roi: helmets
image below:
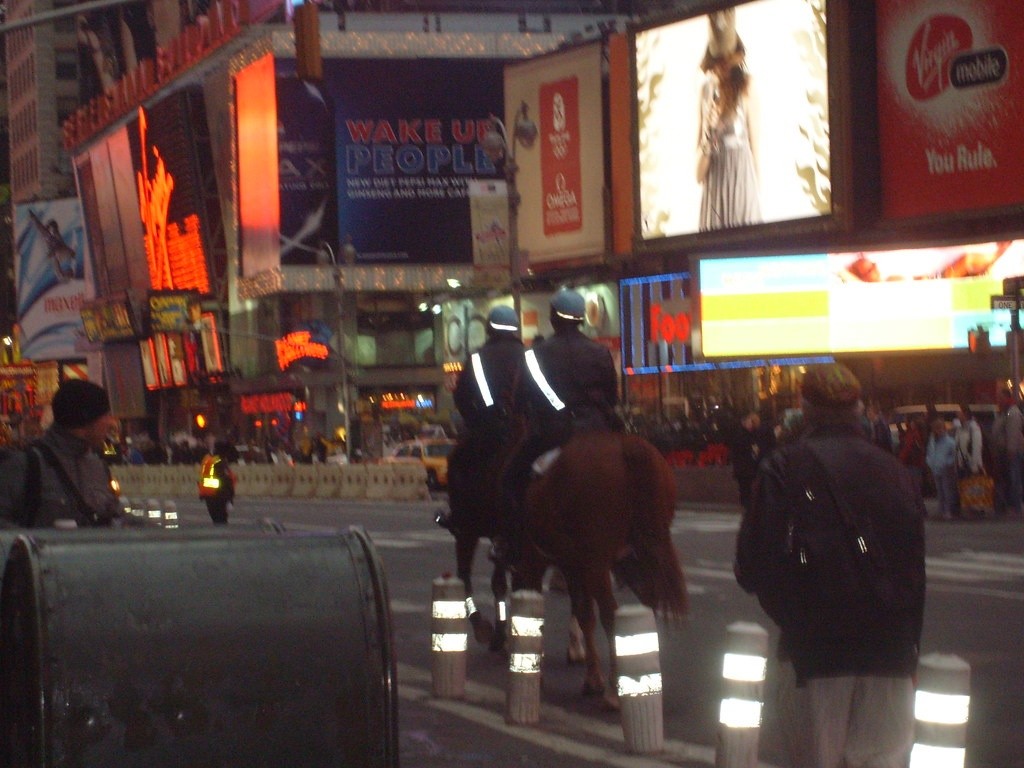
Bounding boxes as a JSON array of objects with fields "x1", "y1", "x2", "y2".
[
  {"x1": 487, "y1": 306, "x2": 519, "y2": 331},
  {"x1": 550, "y1": 291, "x2": 586, "y2": 325}
]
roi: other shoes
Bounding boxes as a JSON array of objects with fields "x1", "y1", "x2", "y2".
[{"x1": 488, "y1": 542, "x2": 519, "y2": 566}]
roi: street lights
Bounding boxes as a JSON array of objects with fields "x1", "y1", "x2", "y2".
[
  {"x1": 319, "y1": 239, "x2": 351, "y2": 464},
  {"x1": 480, "y1": 100, "x2": 539, "y2": 345}
]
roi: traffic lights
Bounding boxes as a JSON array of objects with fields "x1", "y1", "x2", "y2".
[{"x1": 969, "y1": 331, "x2": 977, "y2": 352}]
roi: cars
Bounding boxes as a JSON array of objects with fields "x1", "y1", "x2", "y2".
[{"x1": 378, "y1": 439, "x2": 457, "y2": 487}]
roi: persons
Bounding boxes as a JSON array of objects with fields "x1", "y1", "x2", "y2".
[
  {"x1": 696, "y1": 7, "x2": 760, "y2": 231},
  {"x1": 486, "y1": 297, "x2": 618, "y2": 564},
  {"x1": 198, "y1": 432, "x2": 235, "y2": 525},
  {"x1": 732, "y1": 360, "x2": 926, "y2": 768},
  {"x1": 433, "y1": 304, "x2": 525, "y2": 545},
  {"x1": 734, "y1": 390, "x2": 1024, "y2": 517},
  {"x1": 1, "y1": 378, "x2": 120, "y2": 530}
]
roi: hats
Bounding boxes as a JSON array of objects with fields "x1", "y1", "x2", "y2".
[
  {"x1": 800, "y1": 361, "x2": 861, "y2": 408},
  {"x1": 51, "y1": 379, "x2": 109, "y2": 431}
]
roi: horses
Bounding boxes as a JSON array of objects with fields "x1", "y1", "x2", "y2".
[{"x1": 446, "y1": 334, "x2": 690, "y2": 712}]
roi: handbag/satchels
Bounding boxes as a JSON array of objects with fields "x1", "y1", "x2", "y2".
[{"x1": 960, "y1": 478, "x2": 994, "y2": 513}]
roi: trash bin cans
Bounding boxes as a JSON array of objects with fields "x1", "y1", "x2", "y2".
[{"x1": 0, "y1": 525, "x2": 401, "y2": 768}]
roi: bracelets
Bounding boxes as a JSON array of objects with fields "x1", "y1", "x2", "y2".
[{"x1": 701, "y1": 130, "x2": 714, "y2": 157}]
